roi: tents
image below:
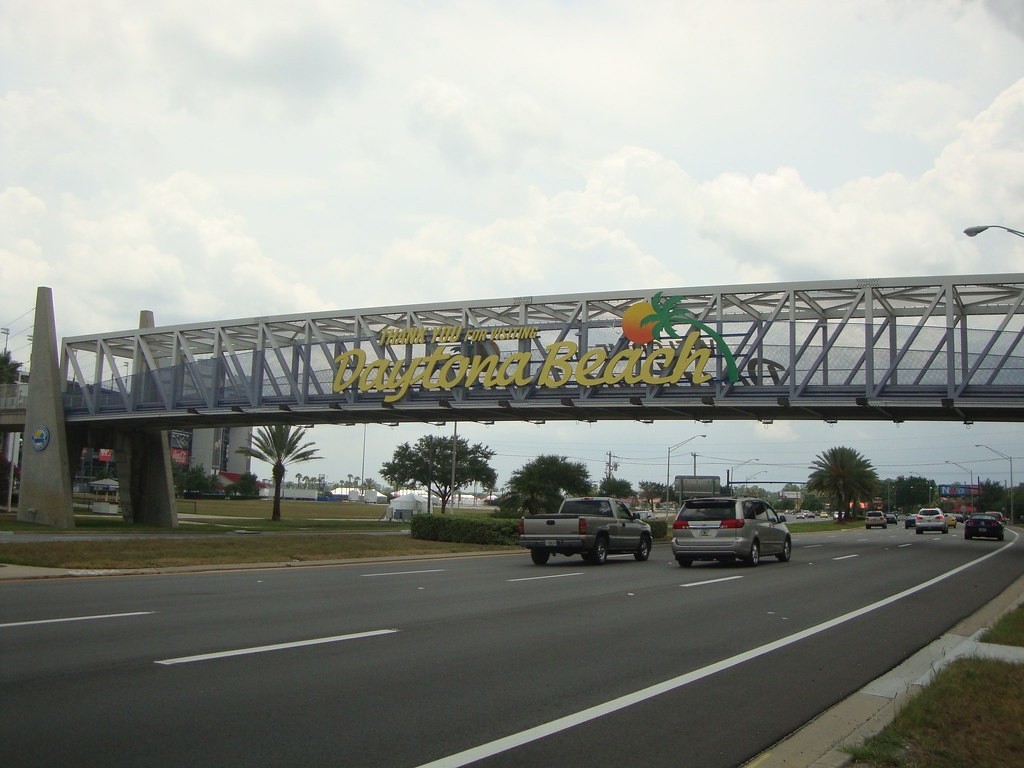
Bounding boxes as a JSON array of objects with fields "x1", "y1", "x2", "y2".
[{"x1": 388, "y1": 493, "x2": 432, "y2": 522}]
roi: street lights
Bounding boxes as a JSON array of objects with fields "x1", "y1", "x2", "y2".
[
  {"x1": 975, "y1": 444, "x2": 1013, "y2": 526},
  {"x1": 908, "y1": 471, "x2": 932, "y2": 505},
  {"x1": 745, "y1": 470, "x2": 767, "y2": 491},
  {"x1": 946, "y1": 458, "x2": 974, "y2": 516},
  {"x1": 665, "y1": 434, "x2": 707, "y2": 519},
  {"x1": 730, "y1": 458, "x2": 760, "y2": 498}
]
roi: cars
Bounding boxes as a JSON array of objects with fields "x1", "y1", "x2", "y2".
[
  {"x1": 964, "y1": 512, "x2": 1006, "y2": 540},
  {"x1": 795, "y1": 507, "x2": 964, "y2": 534}
]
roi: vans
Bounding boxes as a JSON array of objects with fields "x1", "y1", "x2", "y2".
[
  {"x1": 986, "y1": 511, "x2": 1005, "y2": 528},
  {"x1": 671, "y1": 494, "x2": 792, "y2": 567}
]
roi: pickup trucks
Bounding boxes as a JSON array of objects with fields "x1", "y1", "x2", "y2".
[{"x1": 517, "y1": 496, "x2": 653, "y2": 564}]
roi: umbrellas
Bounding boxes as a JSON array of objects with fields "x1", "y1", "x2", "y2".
[{"x1": 87, "y1": 477, "x2": 119, "y2": 502}]
